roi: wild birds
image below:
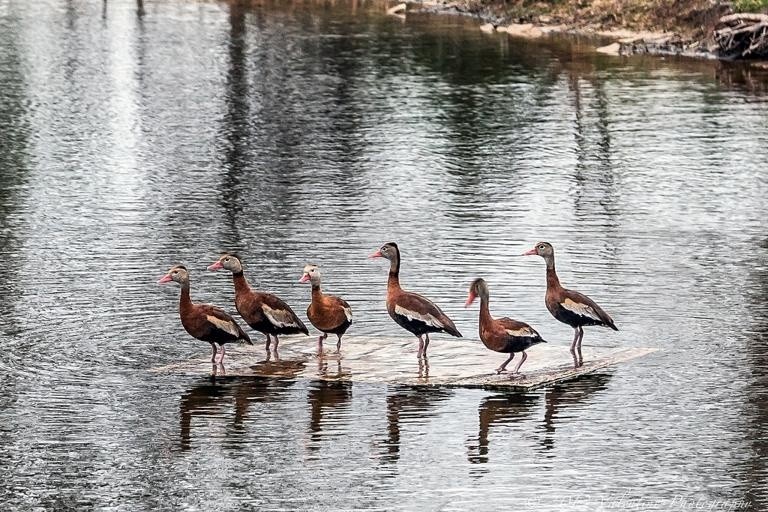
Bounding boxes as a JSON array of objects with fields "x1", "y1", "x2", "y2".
[
  {"x1": 367, "y1": 242, "x2": 463, "y2": 358},
  {"x1": 521, "y1": 242, "x2": 619, "y2": 350},
  {"x1": 157, "y1": 265, "x2": 254, "y2": 362},
  {"x1": 298, "y1": 266, "x2": 353, "y2": 352},
  {"x1": 464, "y1": 277, "x2": 547, "y2": 374},
  {"x1": 206, "y1": 254, "x2": 310, "y2": 352}
]
